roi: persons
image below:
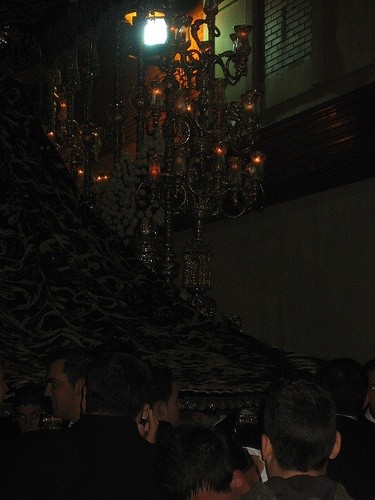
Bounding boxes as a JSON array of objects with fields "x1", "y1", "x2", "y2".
[
  {"x1": 0, "y1": 348, "x2": 178, "y2": 500},
  {"x1": 239, "y1": 374, "x2": 354, "y2": 500},
  {"x1": 317, "y1": 357, "x2": 375, "y2": 500},
  {"x1": 363, "y1": 358, "x2": 375, "y2": 423},
  {"x1": 0, "y1": 362, "x2": 9, "y2": 402},
  {"x1": 158, "y1": 426, "x2": 259, "y2": 500},
  {"x1": 42, "y1": 348, "x2": 86, "y2": 421},
  {"x1": 141, "y1": 366, "x2": 180, "y2": 445},
  {"x1": 13, "y1": 382, "x2": 52, "y2": 432}
]
